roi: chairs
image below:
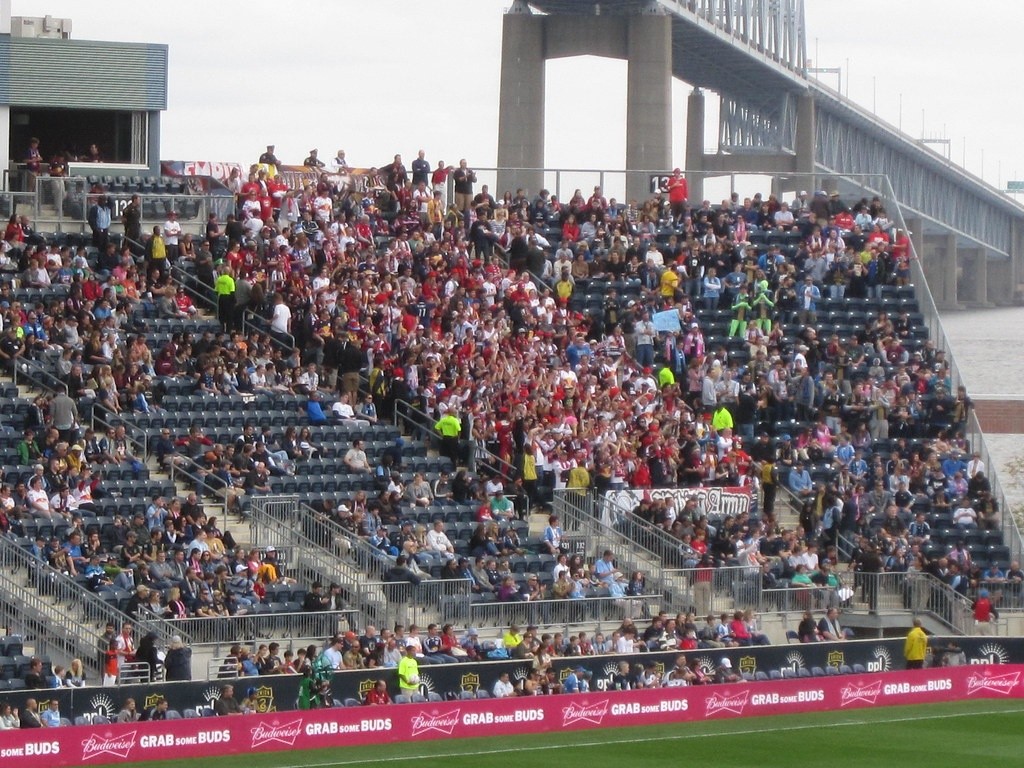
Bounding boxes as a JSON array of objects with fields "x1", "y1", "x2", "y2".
[{"x1": 0, "y1": 170, "x2": 1024, "y2": 730}]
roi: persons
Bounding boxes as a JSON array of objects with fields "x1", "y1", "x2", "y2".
[{"x1": 0, "y1": 143, "x2": 1022, "y2": 733}]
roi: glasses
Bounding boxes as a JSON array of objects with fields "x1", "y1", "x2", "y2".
[
  {"x1": 163, "y1": 433, "x2": 170, "y2": 435},
  {"x1": 139, "y1": 518, "x2": 145, "y2": 520}
]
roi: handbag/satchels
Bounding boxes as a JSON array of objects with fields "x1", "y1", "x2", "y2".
[{"x1": 155, "y1": 358, "x2": 172, "y2": 375}]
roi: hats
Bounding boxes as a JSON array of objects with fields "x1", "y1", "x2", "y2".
[
  {"x1": 236, "y1": 565, "x2": 248, "y2": 573},
  {"x1": 81, "y1": 464, "x2": 93, "y2": 470},
  {"x1": 71, "y1": 445, "x2": 83, "y2": 451},
  {"x1": 265, "y1": 546, "x2": 278, "y2": 552},
  {"x1": 783, "y1": 435, "x2": 791, "y2": 440},
  {"x1": 417, "y1": 325, "x2": 427, "y2": 330},
  {"x1": 830, "y1": 191, "x2": 839, "y2": 196},
  {"x1": 576, "y1": 667, "x2": 587, "y2": 671},
  {"x1": 467, "y1": 628, "x2": 478, "y2": 636},
  {"x1": 799, "y1": 345, "x2": 809, "y2": 351},
  {"x1": 798, "y1": 191, "x2": 808, "y2": 196},
  {"x1": 137, "y1": 585, "x2": 149, "y2": 592},
  {"x1": 2, "y1": 301, "x2": 11, "y2": 308},
  {"x1": 722, "y1": 658, "x2": 732, "y2": 668},
  {"x1": 818, "y1": 191, "x2": 827, "y2": 196},
  {"x1": 126, "y1": 531, "x2": 139, "y2": 536},
  {"x1": 310, "y1": 148, "x2": 318, "y2": 153}
]
